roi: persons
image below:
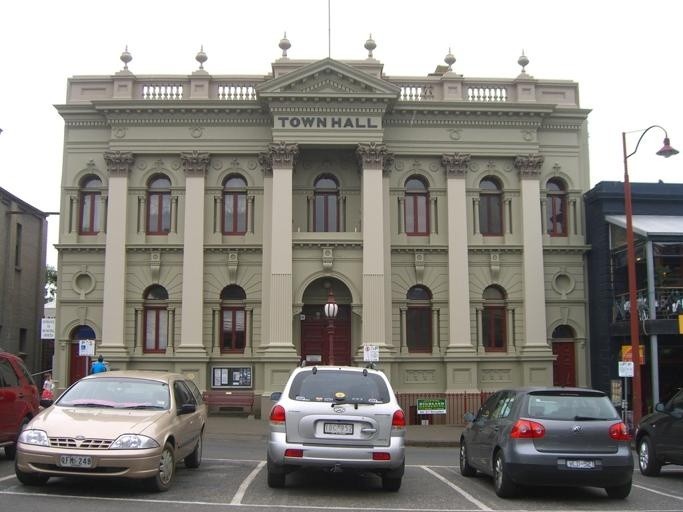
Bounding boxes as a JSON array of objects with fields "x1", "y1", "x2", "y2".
[
  {"x1": 90, "y1": 354, "x2": 107, "y2": 374},
  {"x1": 41, "y1": 373, "x2": 55, "y2": 400}
]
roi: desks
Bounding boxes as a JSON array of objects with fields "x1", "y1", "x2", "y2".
[{"x1": 624, "y1": 298, "x2": 659, "y2": 319}]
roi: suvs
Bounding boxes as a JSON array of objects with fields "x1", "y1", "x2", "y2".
[
  {"x1": 267, "y1": 359, "x2": 408, "y2": 490},
  {"x1": 0, "y1": 350, "x2": 37, "y2": 460}
]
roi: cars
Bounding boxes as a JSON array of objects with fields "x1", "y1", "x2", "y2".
[
  {"x1": 457, "y1": 388, "x2": 633, "y2": 501},
  {"x1": 637, "y1": 389, "x2": 683, "y2": 478},
  {"x1": 14, "y1": 369, "x2": 209, "y2": 494}
]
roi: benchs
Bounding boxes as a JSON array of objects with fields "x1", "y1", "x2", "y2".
[{"x1": 202, "y1": 391, "x2": 255, "y2": 414}]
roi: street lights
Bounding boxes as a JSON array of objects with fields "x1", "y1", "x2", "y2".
[
  {"x1": 321, "y1": 292, "x2": 341, "y2": 365},
  {"x1": 621, "y1": 123, "x2": 681, "y2": 436}
]
